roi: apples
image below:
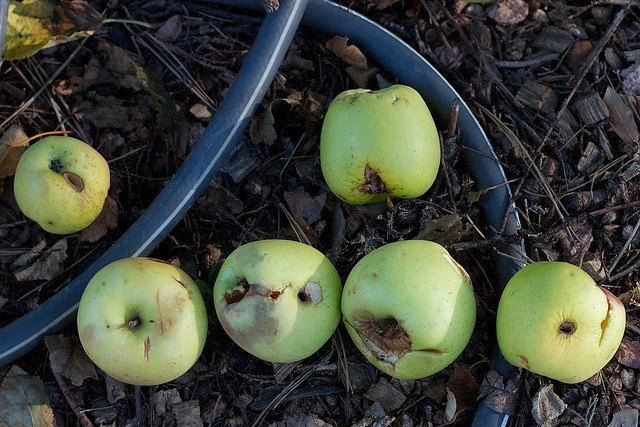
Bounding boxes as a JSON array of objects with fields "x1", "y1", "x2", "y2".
[
  {"x1": 212, "y1": 239, "x2": 344, "y2": 363},
  {"x1": 496, "y1": 260, "x2": 627, "y2": 385},
  {"x1": 340, "y1": 239, "x2": 477, "y2": 380},
  {"x1": 77, "y1": 258, "x2": 208, "y2": 386},
  {"x1": 319, "y1": 84, "x2": 441, "y2": 205},
  {"x1": 14, "y1": 136, "x2": 111, "y2": 235}
]
